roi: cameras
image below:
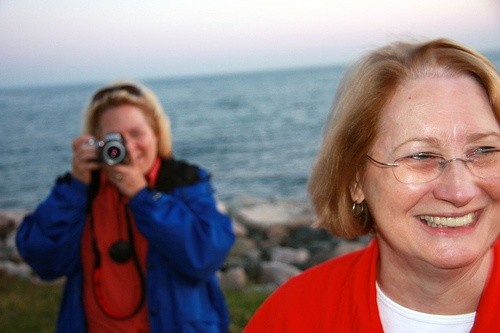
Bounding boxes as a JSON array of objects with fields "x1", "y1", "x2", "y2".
[{"x1": 84, "y1": 132, "x2": 130, "y2": 167}]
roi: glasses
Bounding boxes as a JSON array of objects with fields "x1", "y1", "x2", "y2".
[
  {"x1": 363, "y1": 147, "x2": 500, "y2": 184},
  {"x1": 91, "y1": 86, "x2": 141, "y2": 105}
]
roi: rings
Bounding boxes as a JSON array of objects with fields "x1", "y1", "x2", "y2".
[{"x1": 114, "y1": 172, "x2": 121, "y2": 180}]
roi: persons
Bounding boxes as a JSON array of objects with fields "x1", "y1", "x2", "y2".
[
  {"x1": 240, "y1": 36, "x2": 500, "y2": 333},
  {"x1": 15, "y1": 82, "x2": 235, "y2": 333}
]
